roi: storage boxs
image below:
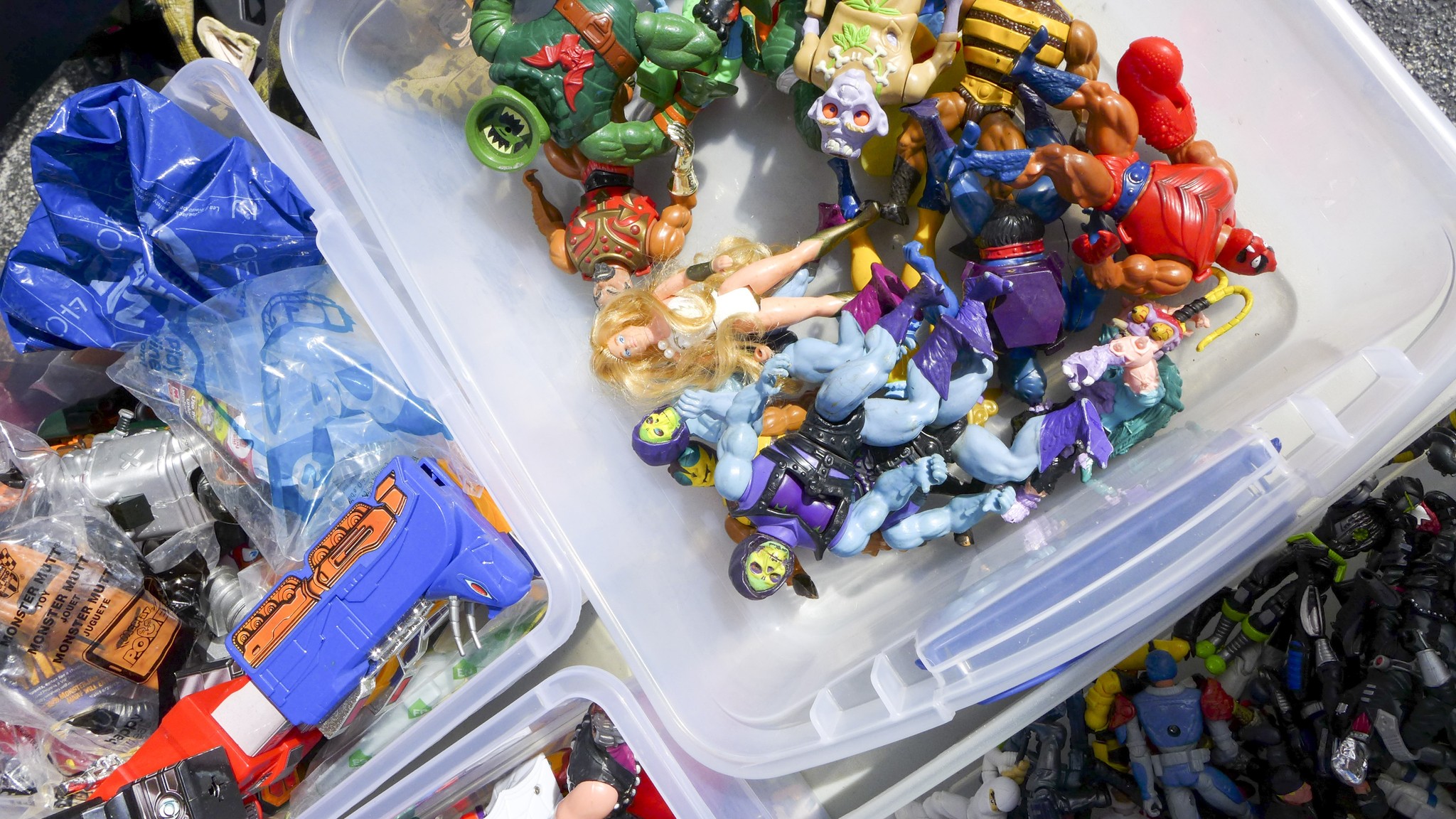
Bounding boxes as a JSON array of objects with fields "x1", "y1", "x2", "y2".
[{"x1": 0, "y1": 0, "x2": 1456, "y2": 819}]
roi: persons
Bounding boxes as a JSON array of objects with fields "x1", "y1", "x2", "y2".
[
  {"x1": 895, "y1": 411, "x2": 1456, "y2": 819},
  {"x1": 471, "y1": 1, "x2": 1278, "y2": 601}
]
